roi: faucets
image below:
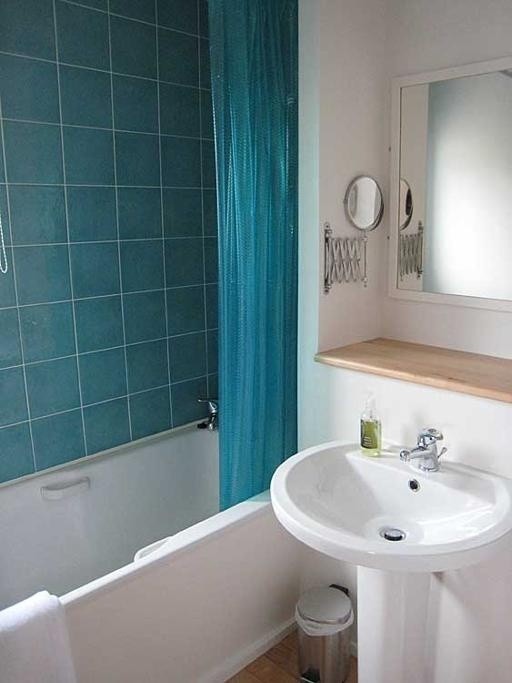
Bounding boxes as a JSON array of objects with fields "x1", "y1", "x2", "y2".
[
  {"x1": 197, "y1": 398, "x2": 219, "y2": 432},
  {"x1": 401, "y1": 428, "x2": 443, "y2": 471}
]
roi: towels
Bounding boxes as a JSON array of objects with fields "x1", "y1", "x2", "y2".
[{"x1": 0, "y1": 586, "x2": 81, "y2": 683}]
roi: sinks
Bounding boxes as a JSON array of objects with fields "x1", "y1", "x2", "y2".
[{"x1": 270, "y1": 437, "x2": 512, "y2": 574}]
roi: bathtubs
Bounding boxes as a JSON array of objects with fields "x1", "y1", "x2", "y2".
[{"x1": 0, "y1": 413, "x2": 299, "y2": 683}]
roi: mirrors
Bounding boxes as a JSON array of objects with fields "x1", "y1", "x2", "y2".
[
  {"x1": 386, "y1": 51, "x2": 511, "y2": 308},
  {"x1": 399, "y1": 177, "x2": 427, "y2": 283},
  {"x1": 321, "y1": 174, "x2": 387, "y2": 296}
]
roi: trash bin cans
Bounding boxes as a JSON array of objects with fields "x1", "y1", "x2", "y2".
[{"x1": 296, "y1": 585, "x2": 355, "y2": 683}]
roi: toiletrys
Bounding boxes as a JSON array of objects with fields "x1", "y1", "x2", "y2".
[{"x1": 360, "y1": 395, "x2": 382, "y2": 457}]
